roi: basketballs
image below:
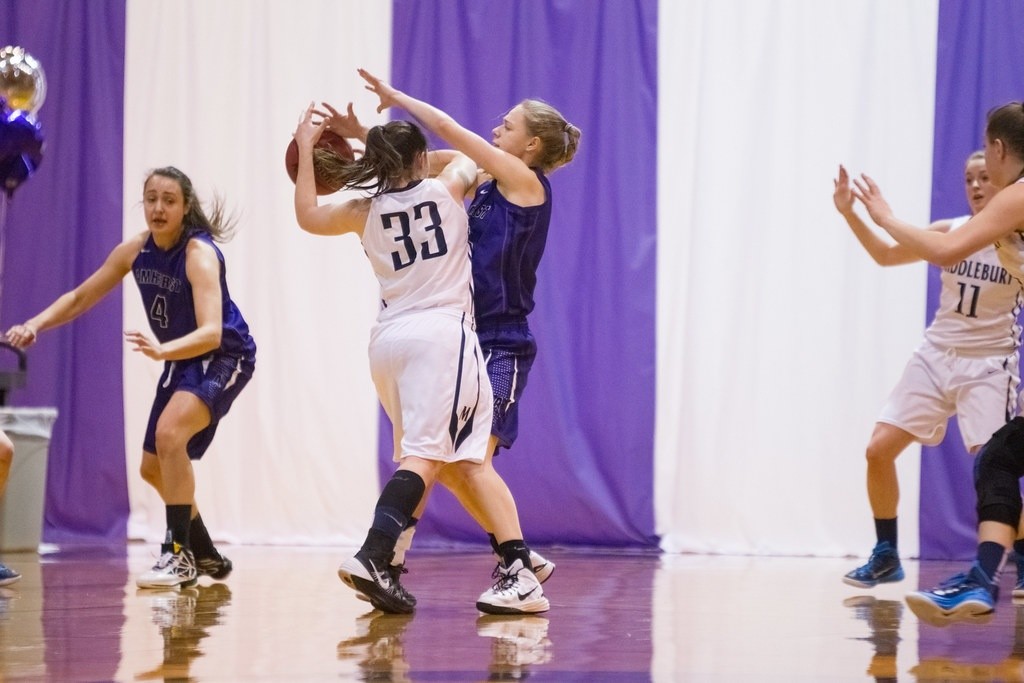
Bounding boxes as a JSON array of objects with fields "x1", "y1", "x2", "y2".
[{"x1": 284, "y1": 128, "x2": 355, "y2": 199}]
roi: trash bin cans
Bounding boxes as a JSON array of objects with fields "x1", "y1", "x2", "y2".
[{"x1": 0, "y1": 406, "x2": 58, "y2": 552}]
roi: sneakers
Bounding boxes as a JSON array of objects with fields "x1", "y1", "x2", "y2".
[
  {"x1": 1012, "y1": 551, "x2": 1024, "y2": 597},
  {"x1": 905, "y1": 560, "x2": 1001, "y2": 628},
  {"x1": 1, "y1": 565, "x2": 21, "y2": 587},
  {"x1": 181, "y1": 553, "x2": 233, "y2": 589},
  {"x1": 843, "y1": 541, "x2": 905, "y2": 589},
  {"x1": 136, "y1": 543, "x2": 199, "y2": 590},
  {"x1": 338, "y1": 551, "x2": 417, "y2": 614},
  {"x1": 354, "y1": 592, "x2": 370, "y2": 601},
  {"x1": 475, "y1": 558, "x2": 550, "y2": 615},
  {"x1": 529, "y1": 550, "x2": 556, "y2": 583}
]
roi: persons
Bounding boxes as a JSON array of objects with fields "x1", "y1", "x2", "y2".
[
  {"x1": 290, "y1": 102, "x2": 493, "y2": 613},
  {"x1": 0, "y1": 428, "x2": 22, "y2": 587},
  {"x1": 310, "y1": 67, "x2": 581, "y2": 614},
  {"x1": 5, "y1": 166, "x2": 256, "y2": 589},
  {"x1": 852, "y1": 101, "x2": 1024, "y2": 625},
  {"x1": 832, "y1": 148, "x2": 1023, "y2": 598}
]
